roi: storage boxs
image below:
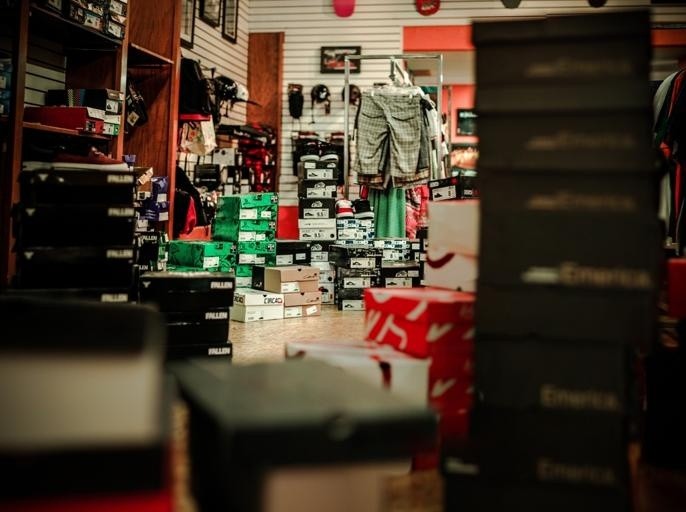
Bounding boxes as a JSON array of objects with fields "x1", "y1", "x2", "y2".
[
  {"x1": 440, "y1": 6, "x2": 657, "y2": 512},
  {"x1": 23, "y1": 88, "x2": 125, "y2": 138},
  {"x1": 0, "y1": 160, "x2": 480, "y2": 512},
  {"x1": 42, "y1": 0, "x2": 128, "y2": 41}
]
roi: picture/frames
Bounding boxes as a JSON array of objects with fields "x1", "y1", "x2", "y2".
[
  {"x1": 179, "y1": 0, "x2": 240, "y2": 48},
  {"x1": 321, "y1": 46, "x2": 361, "y2": 73}
]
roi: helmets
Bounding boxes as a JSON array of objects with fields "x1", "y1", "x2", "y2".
[
  {"x1": 311, "y1": 85, "x2": 330, "y2": 102},
  {"x1": 342, "y1": 85, "x2": 360, "y2": 103},
  {"x1": 215, "y1": 76, "x2": 237, "y2": 99},
  {"x1": 237, "y1": 85, "x2": 249, "y2": 101}
]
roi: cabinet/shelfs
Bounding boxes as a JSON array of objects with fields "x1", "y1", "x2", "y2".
[{"x1": 0, "y1": 0, "x2": 182, "y2": 290}]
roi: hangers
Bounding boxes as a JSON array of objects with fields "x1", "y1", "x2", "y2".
[{"x1": 360, "y1": 73, "x2": 419, "y2": 103}]
